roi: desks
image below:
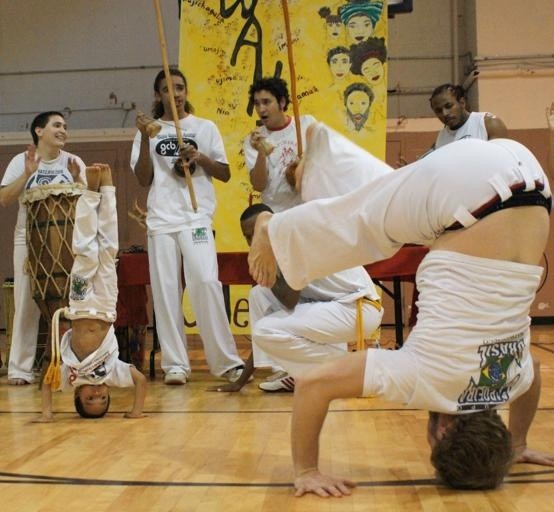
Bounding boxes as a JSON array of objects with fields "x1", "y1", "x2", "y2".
[{"x1": 115, "y1": 247, "x2": 431, "y2": 381}]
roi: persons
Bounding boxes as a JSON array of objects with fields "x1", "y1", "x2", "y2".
[
  {"x1": 206, "y1": 203, "x2": 385, "y2": 392},
  {"x1": 31, "y1": 164, "x2": 148, "y2": 423},
  {"x1": 343, "y1": 83, "x2": 374, "y2": 131},
  {"x1": 129, "y1": 70, "x2": 254, "y2": 384},
  {"x1": 349, "y1": 37, "x2": 386, "y2": 83},
  {"x1": 248, "y1": 121, "x2": 554, "y2": 500},
  {"x1": 0, "y1": 111, "x2": 88, "y2": 385},
  {"x1": 341, "y1": 0, "x2": 383, "y2": 42},
  {"x1": 327, "y1": 46, "x2": 352, "y2": 79},
  {"x1": 318, "y1": 7, "x2": 342, "y2": 37},
  {"x1": 429, "y1": 84, "x2": 507, "y2": 146},
  {"x1": 241, "y1": 77, "x2": 318, "y2": 211}
]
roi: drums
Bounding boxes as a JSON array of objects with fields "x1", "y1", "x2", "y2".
[{"x1": 22, "y1": 183, "x2": 82, "y2": 369}]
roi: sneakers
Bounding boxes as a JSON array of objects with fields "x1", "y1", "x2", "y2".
[
  {"x1": 258, "y1": 370, "x2": 294, "y2": 392},
  {"x1": 164, "y1": 372, "x2": 191, "y2": 385},
  {"x1": 222, "y1": 364, "x2": 254, "y2": 384}
]
